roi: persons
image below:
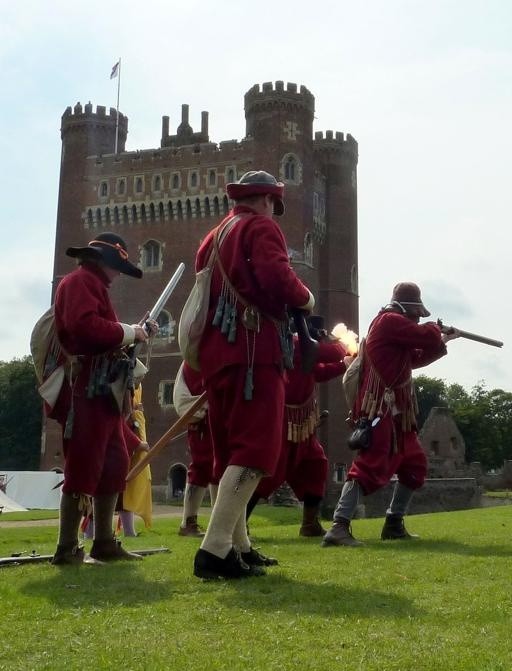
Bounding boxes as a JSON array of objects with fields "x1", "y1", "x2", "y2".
[
  {"x1": 179, "y1": 360, "x2": 218, "y2": 537},
  {"x1": 195, "y1": 169, "x2": 316, "y2": 581},
  {"x1": 247, "y1": 316, "x2": 358, "y2": 538},
  {"x1": 322, "y1": 281, "x2": 459, "y2": 547},
  {"x1": 46, "y1": 232, "x2": 158, "y2": 565},
  {"x1": 80, "y1": 358, "x2": 153, "y2": 538}
]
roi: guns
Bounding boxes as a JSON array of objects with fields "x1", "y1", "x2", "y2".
[
  {"x1": 293, "y1": 306, "x2": 318, "y2": 373},
  {"x1": 437, "y1": 318, "x2": 504, "y2": 349},
  {"x1": 0, "y1": 548, "x2": 169, "y2": 566},
  {"x1": 107, "y1": 262, "x2": 185, "y2": 382}
]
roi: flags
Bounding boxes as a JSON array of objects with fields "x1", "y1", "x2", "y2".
[{"x1": 110, "y1": 61, "x2": 120, "y2": 79}]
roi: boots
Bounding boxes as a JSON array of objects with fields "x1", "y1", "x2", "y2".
[
  {"x1": 323, "y1": 521, "x2": 361, "y2": 545},
  {"x1": 382, "y1": 515, "x2": 419, "y2": 539},
  {"x1": 299, "y1": 499, "x2": 326, "y2": 535}
]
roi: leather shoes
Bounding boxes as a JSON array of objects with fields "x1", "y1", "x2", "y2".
[
  {"x1": 178, "y1": 515, "x2": 204, "y2": 537},
  {"x1": 91, "y1": 536, "x2": 141, "y2": 561},
  {"x1": 241, "y1": 547, "x2": 276, "y2": 565},
  {"x1": 193, "y1": 544, "x2": 264, "y2": 580},
  {"x1": 52, "y1": 540, "x2": 102, "y2": 566}
]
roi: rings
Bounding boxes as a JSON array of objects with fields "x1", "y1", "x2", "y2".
[{"x1": 155, "y1": 324, "x2": 158, "y2": 327}]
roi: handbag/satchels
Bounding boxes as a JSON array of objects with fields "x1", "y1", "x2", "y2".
[
  {"x1": 30, "y1": 304, "x2": 56, "y2": 385},
  {"x1": 172, "y1": 360, "x2": 208, "y2": 423},
  {"x1": 177, "y1": 214, "x2": 240, "y2": 371},
  {"x1": 341, "y1": 335, "x2": 364, "y2": 407}
]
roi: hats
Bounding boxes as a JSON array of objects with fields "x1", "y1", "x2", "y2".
[
  {"x1": 386, "y1": 283, "x2": 430, "y2": 316},
  {"x1": 227, "y1": 169, "x2": 285, "y2": 216},
  {"x1": 66, "y1": 231, "x2": 143, "y2": 278}
]
